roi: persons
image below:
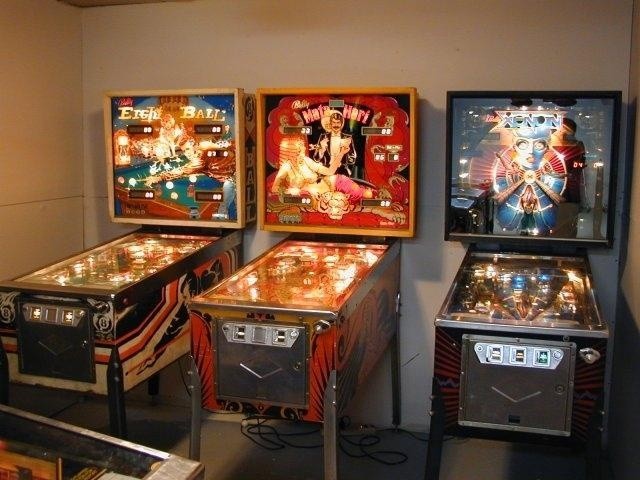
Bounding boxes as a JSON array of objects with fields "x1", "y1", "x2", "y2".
[
  {"x1": 272, "y1": 136, "x2": 362, "y2": 207},
  {"x1": 516, "y1": 184, "x2": 540, "y2": 236},
  {"x1": 153, "y1": 115, "x2": 177, "y2": 159},
  {"x1": 313, "y1": 113, "x2": 357, "y2": 180}
]
